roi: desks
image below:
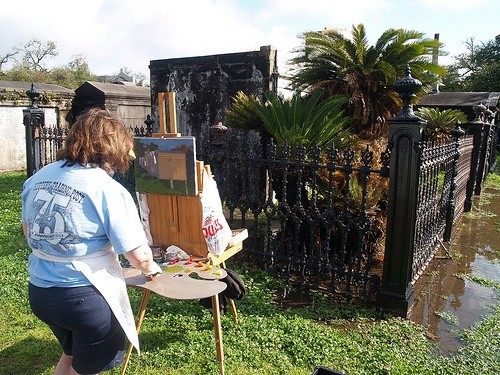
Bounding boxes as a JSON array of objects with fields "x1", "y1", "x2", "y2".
[{"x1": 122, "y1": 238, "x2": 243, "y2": 375}]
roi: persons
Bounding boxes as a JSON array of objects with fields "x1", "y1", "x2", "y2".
[{"x1": 20, "y1": 108, "x2": 162, "y2": 375}]
